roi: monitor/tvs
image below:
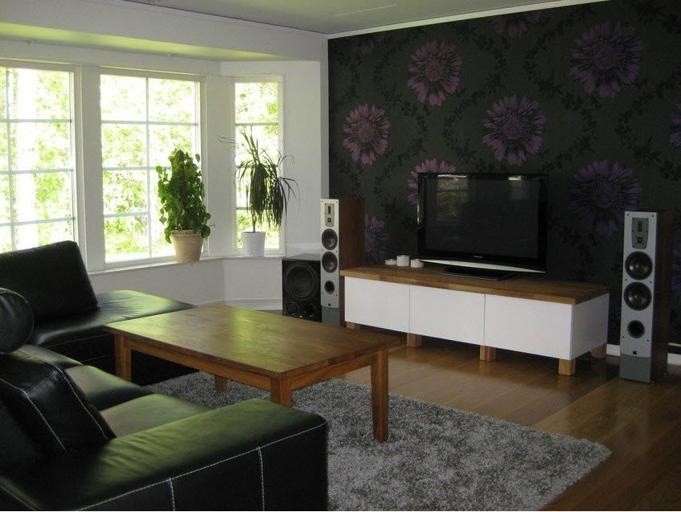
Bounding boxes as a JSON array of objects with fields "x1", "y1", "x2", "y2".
[{"x1": 416, "y1": 172, "x2": 549, "y2": 281}]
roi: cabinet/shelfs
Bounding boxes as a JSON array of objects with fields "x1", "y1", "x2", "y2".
[{"x1": 338, "y1": 264, "x2": 610, "y2": 360}]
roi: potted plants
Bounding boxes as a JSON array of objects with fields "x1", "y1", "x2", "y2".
[
  {"x1": 156, "y1": 149, "x2": 211, "y2": 264},
  {"x1": 234, "y1": 125, "x2": 301, "y2": 256}
]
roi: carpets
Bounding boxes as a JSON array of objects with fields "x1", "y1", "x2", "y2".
[{"x1": 145, "y1": 371, "x2": 613, "y2": 511}]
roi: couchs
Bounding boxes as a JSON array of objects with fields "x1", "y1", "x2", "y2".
[
  {"x1": 0, "y1": 240, "x2": 198, "y2": 388},
  {"x1": 0, "y1": 286, "x2": 330, "y2": 511}
]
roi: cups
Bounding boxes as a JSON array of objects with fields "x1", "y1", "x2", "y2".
[{"x1": 396, "y1": 255, "x2": 410, "y2": 267}]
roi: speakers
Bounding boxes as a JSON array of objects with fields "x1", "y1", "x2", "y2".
[
  {"x1": 619, "y1": 208, "x2": 671, "y2": 384},
  {"x1": 282, "y1": 254, "x2": 322, "y2": 322},
  {"x1": 320, "y1": 199, "x2": 344, "y2": 327}
]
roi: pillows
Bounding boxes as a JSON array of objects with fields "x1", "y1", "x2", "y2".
[{"x1": 0, "y1": 242, "x2": 99, "y2": 328}]
